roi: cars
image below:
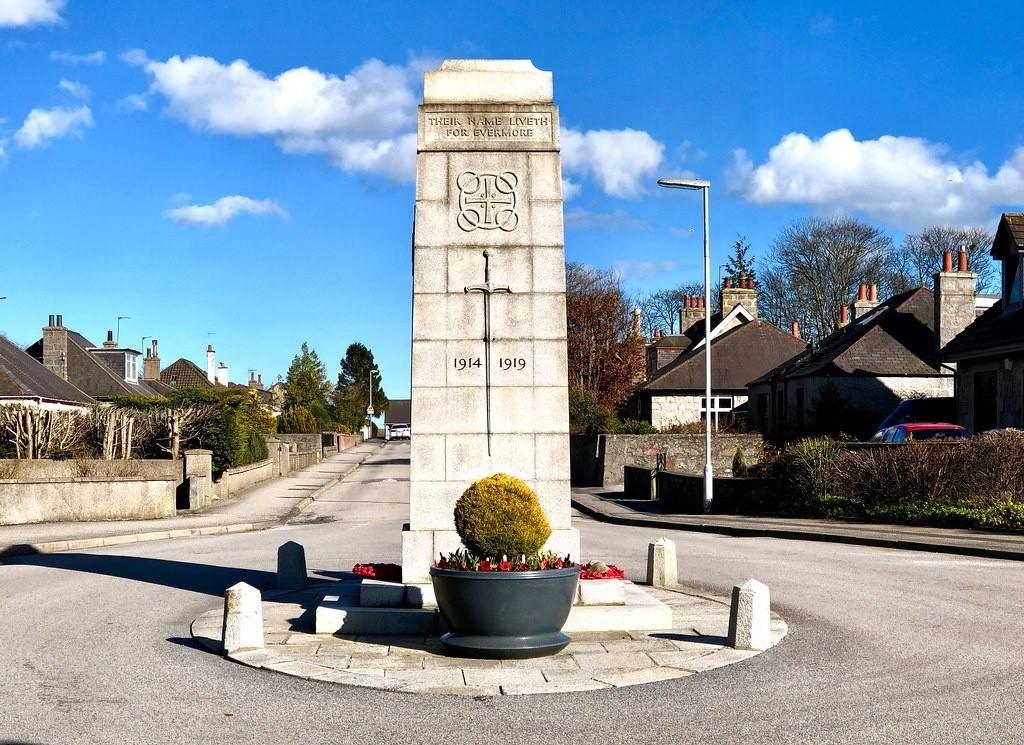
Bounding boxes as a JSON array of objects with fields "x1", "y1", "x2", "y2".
[
  {"x1": 878, "y1": 396, "x2": 958, "y2": 430},
  {"x1": 870, "y1": 423, "x2": 973, "y2": 442},
  {"x1": 388, "y1": 424, "x2": 411, "y2": 441}
]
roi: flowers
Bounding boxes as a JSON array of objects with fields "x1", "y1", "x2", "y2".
[{"x1": 433, "y1": 471, "x2": 577, "y2": 573}]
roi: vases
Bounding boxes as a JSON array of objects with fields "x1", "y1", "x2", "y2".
[{"x1": 429, "y1": 565, "x2": 579, "y2": 660}]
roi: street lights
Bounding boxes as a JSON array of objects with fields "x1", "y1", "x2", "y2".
[
  {"x1": 657, "y1": 178, "x2": 713, "y2": 512},
  {"x1": 369, "y1": 370, "x2": 378, "y2": 438}
]
